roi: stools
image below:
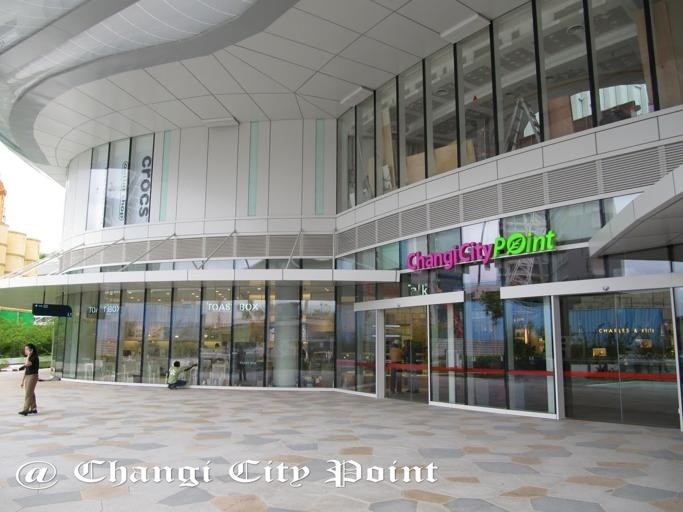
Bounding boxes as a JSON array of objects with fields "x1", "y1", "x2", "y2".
[{"x1": 84, "y1": 362, "x2": 227, "y2": 386}]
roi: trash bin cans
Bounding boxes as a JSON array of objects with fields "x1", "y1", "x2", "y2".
[{"x1": 132, "y1": 375, "x2": 141, "y2": 383}]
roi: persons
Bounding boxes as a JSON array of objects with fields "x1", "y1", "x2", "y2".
[
  {"x1": 168, "y1": 361, "x2": 198, "y2": 389},
  {"x1": 214, "y1": 343, "x2": 226, "y2": 353},
  {"x1": 12, "y1": 343, "x2": 39, "y2": 416},
  {"x1": 389, "y1": 339, "x2": 406, "y2": 394},
  {"x1": 236, "y1": 345, "x2": 247, "y2": 382}
]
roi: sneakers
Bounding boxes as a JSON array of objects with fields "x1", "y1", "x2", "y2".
[
  {"x1": 28, "y1": 410, "x2": 38, "y2": 414},
  {"x1": 18, "y1": 409, "x2": 29, "y2": 416}
]
